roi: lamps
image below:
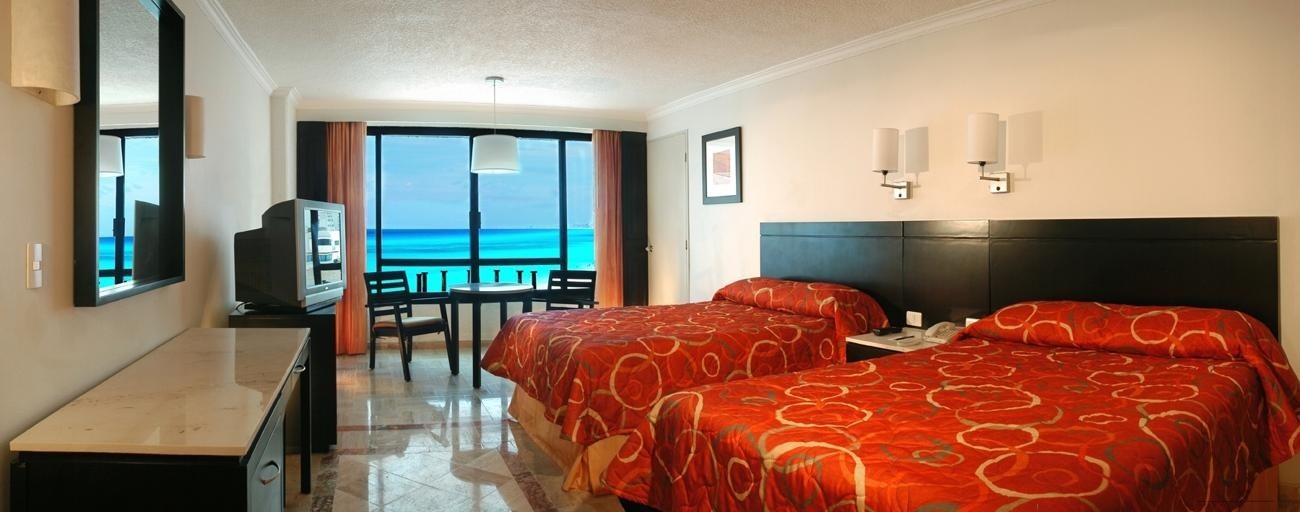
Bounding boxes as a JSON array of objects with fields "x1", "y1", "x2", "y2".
[
  {"x1": 869, "y1": 125, "x2": 913, "y2": 201},
  {"x1": 97, "y1": 131, "x2": 124, "y2": 183},
  {"x1": 961, "y1": 113, "x2": 1010, "y2": 196},
  {"x1": 185, "y1": 95, "x2": 206, "y2": 161},
  {"x1": 8, "y1": 0, "x2": 80, "y2": 109},
  {"x1": 468, "y1": 74, "x2": 519, "y2": 174}
]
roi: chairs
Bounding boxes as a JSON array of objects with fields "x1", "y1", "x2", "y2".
[
  {"x1": 545, "y1": 268, "x2": 599, "y2": 311},
  {"x1": 363, "y1": 270, "x2": 456, "y2": 382}
]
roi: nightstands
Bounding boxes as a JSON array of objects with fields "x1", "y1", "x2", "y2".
[{"x1": 843, "y1": 327, "x2": 962, "y2": 363}]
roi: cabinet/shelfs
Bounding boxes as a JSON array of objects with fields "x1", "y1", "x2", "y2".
[{"x1": 233, "y1": 300, "x2": 338, "y2": 457}]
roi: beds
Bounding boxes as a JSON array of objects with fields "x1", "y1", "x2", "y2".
[
  {"x1": 480, "y1": 219, "x2": 910, "y2": 510},
  {"x1": 597, "y1": 215, "x2": 1300, "y2": 508}
]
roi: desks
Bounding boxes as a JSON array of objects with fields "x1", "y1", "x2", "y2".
[
  {"x1": 10, "y1": 325, "x2": 317, "y2": 510},
  {"x1": 447, "y1": 282, "x2": 535, "y2": 388}
]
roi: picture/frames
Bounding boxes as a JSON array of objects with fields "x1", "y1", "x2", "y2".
[{"x1": 701, "y1": 125, "x2": 743, "y2": 207}]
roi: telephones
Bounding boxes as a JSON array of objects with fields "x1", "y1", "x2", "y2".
[{"x1": 923, "y1": 321, "x2": 965, "y2": 343}]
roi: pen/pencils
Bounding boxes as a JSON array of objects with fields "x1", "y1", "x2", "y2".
[{"x1": 896, "y1": 335, "x2": 915, "y2": 340}]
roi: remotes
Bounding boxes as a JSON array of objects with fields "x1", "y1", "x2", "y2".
[{"x1": 872, "y1": 326, "x2": 903, "y2": 336}]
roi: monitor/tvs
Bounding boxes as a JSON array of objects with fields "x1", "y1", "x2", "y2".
[
  {"x1": 132, "y1": 200, "x2": 160, "y2": 280},
  {"x1": 233, "y1": 198, "x2": 347, "y2": 314}
]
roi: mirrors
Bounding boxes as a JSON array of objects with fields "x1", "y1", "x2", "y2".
[{"x1": 73, "y1": 0, "x2": 185, "y2": 308}]
